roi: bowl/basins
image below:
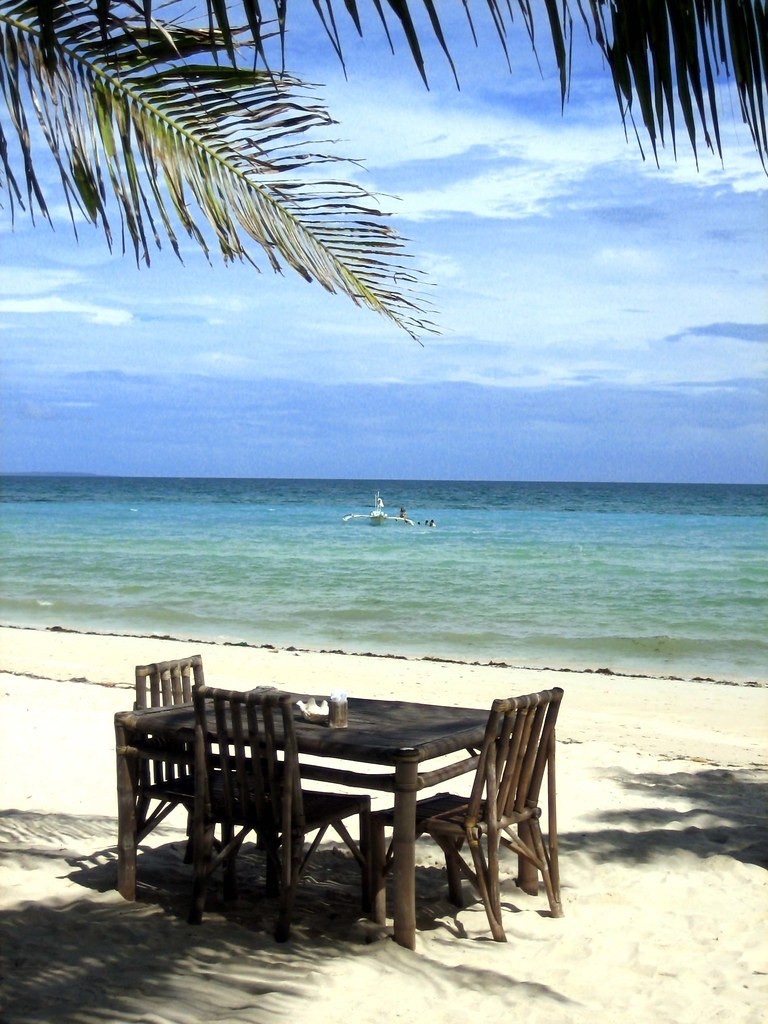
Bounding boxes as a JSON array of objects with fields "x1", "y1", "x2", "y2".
[{"x1": 295, "y1": 697, "x2": 330, "y2": 723}]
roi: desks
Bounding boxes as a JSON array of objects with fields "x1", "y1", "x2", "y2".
[{"x1": 114, "y1": 686, "x2": 538, "y2": 952}]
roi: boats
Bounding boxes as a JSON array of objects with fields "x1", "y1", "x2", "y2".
[{"x1": 342, "y1": 489, "x2": 414, "y2": 526}]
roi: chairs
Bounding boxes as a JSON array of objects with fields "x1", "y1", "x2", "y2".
[
  {"x1": 188, "y1": 683, "x2": 371, "y2": 944},
  {"x1": 371, "y1": 687, "x2": 564, "y2": 942},
  {"x1": 109, "y1": 655, "x2": 281, "y2": 911}
]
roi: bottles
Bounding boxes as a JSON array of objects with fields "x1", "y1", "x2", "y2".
[{"x1": 328, "y1": 700, "x2": 348, "y2": 729}]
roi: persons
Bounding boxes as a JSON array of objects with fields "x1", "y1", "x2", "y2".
[{"x1": 399, "y1": 506, "x2": 437, "y2": 527}]
